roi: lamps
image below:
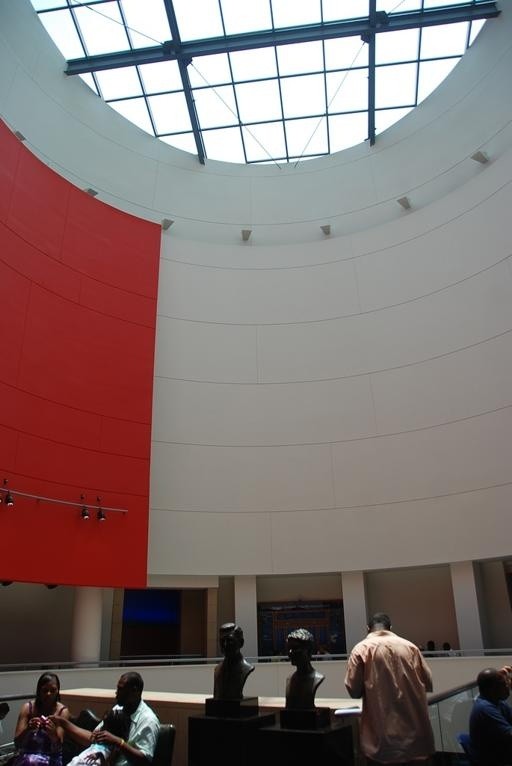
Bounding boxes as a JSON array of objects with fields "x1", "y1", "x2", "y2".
[{"x1": 0, "y1": 488, "x2": 129, "y2": 523}]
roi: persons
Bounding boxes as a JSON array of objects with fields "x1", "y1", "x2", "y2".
[
  {"x1": 316, "y1": 644, "x2": 332, "y2": 661},
  {"x1": 49, "y1": 673, "x2": 161, "y2": 766},
  {"x1": 444, "y1": 642, "x2": 459, "y2": 656},
  {"x1": 9, "y1": 672, "x2": 68, "y2": 765},
  {"x1": 212, "y1": 621, "x2": 255, "y2": 699},
  {"x1": 469, "y1": 668, "x2": 512, "y2": 766},
  {"x1": 422, "y1": 640, "x2": 438, "y2": 657},
  {"x1": 343, "y1": 613, "x2": 435, "y2": 765},
  {"x1": 284, "y1": 628, "x2": 325, "y2": 708},
  {"x1": 68, "y1": 709, "x2": 131, "y2": 766}
]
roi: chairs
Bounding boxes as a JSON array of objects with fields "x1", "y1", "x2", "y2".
[
  {"x1": 61, "y1": 711, "x2": 175, "y2": 765},
  {"x1": 454, "y1": 728, "x2": 469, "y2": 754}
]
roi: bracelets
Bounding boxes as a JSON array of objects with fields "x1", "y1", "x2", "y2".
[{"x1": 119, "y1": 738, "x2": 125, "y2": 748}]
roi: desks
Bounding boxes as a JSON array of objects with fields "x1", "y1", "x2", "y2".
[{"x1": 57, "y1": 686, "x2": 361, "y2": 766}]
